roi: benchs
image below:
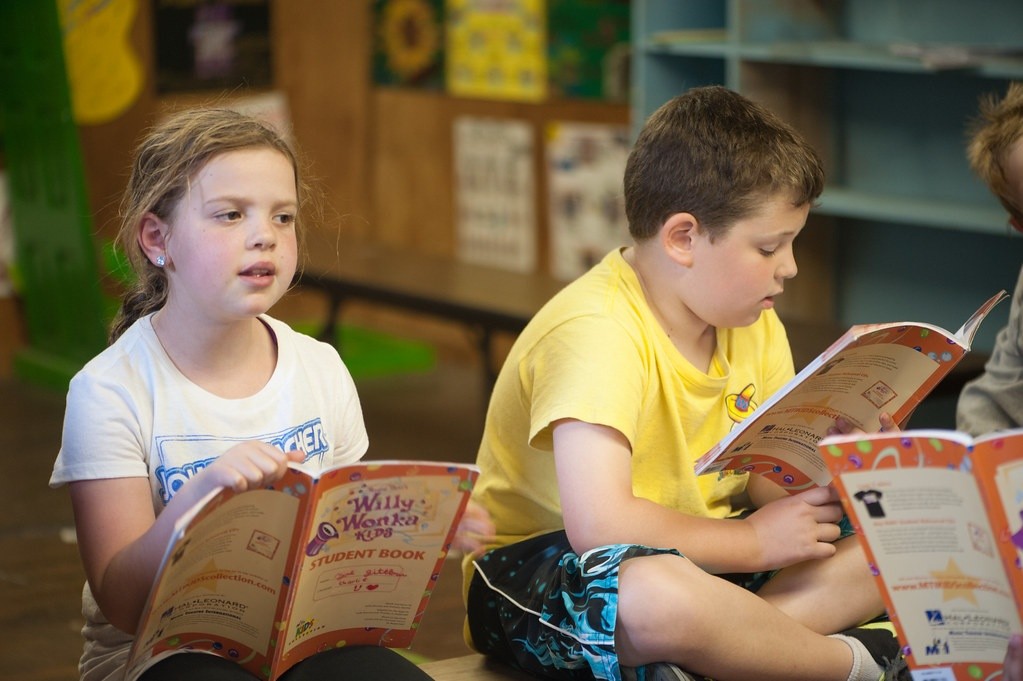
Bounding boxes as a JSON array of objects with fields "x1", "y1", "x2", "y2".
[
  {"x1": 783, "y1": 315, "x2": 984, "y2": 401},
  {"x1": 291, "y1": 227, "x2": 570, "y2": 408}
]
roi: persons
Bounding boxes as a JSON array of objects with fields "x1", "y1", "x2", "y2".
[
  {"x1": 46, "y1": 106, "x2": 494, "y2": 681},
  {"x1": 462, "y1": 85, "x2": 919, "y2": 681},
  {"x1": 955, "y1": 81, "x2": 1023, "y2": 680}
]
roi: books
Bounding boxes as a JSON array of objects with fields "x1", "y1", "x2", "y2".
[
  {"x1": 693, "y1": 290, "x2": 1013, "y2": 498},
  {"x1": 817, "y1": 429, "x2": 1023, "y2": 681},
  {"x1": 124, "y1": 459, "x2": 481, "y2": 681}
]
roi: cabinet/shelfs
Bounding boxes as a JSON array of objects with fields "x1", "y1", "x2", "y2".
[{"x1": 629, "y1": 0, "x2": 1023, "y2": 349}]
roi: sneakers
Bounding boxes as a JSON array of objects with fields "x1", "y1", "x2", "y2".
[
  {"x1": 645, "y1": 663, "x2": 694, "y2": 681},
  {"x1": 842, "y1": 614, "x2": 913, "y2": 681}
]
